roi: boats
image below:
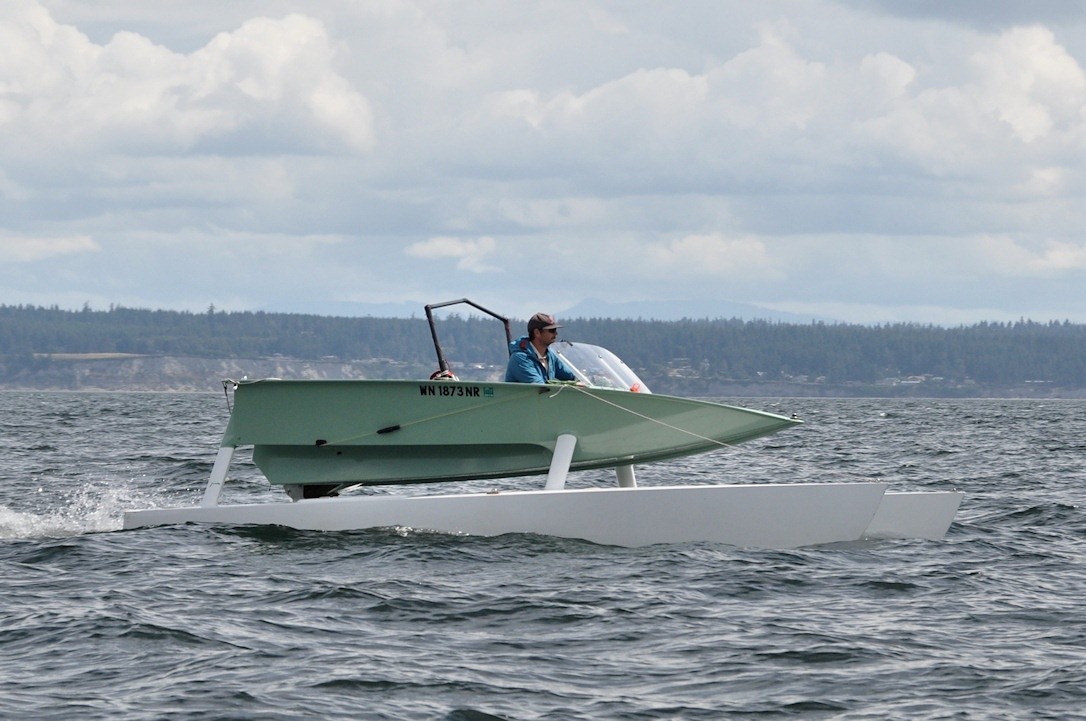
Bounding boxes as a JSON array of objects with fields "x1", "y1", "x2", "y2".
[{"x1": 123, "y1": 299, "x2": 969, "y2": 550}]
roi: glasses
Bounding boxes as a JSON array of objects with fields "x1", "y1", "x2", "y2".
[{"x1": 547, "y1": 328, "x2": 557, "y2": 333}]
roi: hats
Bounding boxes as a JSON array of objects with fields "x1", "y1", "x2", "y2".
[{"x1": 528, "y1": 312, "x2": 564, "y2": 332}]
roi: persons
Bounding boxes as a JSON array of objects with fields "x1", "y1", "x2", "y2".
[{"x1": 504, "y1": 312, "x2": 579, "y2": 384}]
